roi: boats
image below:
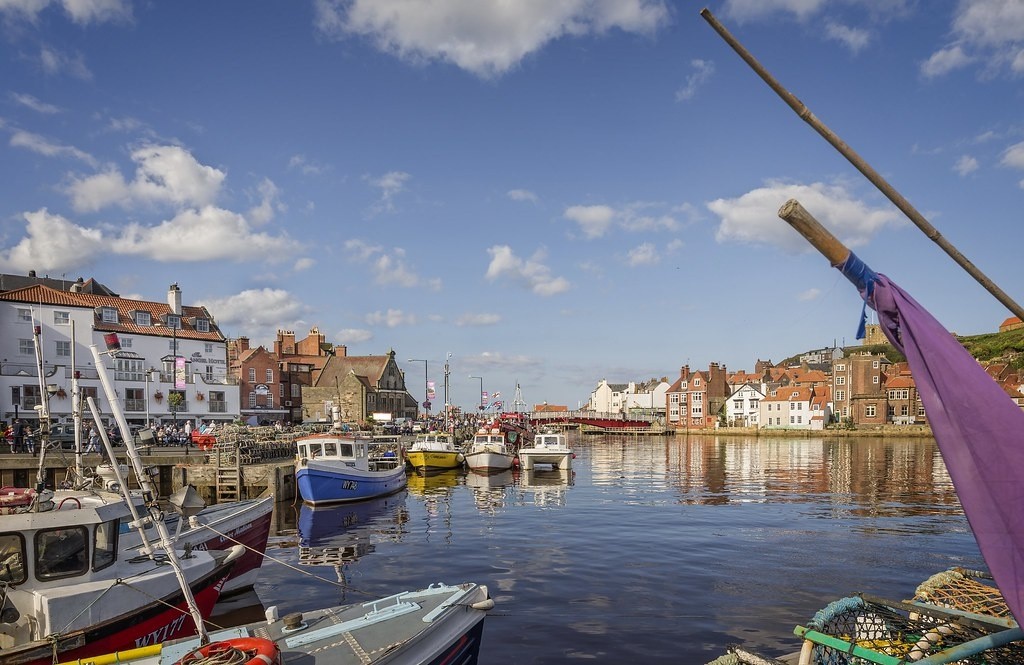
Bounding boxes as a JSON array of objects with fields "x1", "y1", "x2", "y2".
[
  {"x1": 515, "y1": 434, "x2": 574, "y2": 471},
  {"x1": 299, "y1": 487, "x2": 408, "y2": 581},
  {"x1": 26, "y1": 332, "x2": 494, "y2": 665},
  {"x1": 406, "y1": 430, "x2": 459, "y2": 467},
  {"x1": 293, "y1": 407, "x2": 406, "y2": 503},
  {"x1": 522, "y1": 469, "x2": 574, "y2": 505},
  {"x1": 405, "y1": 469, "x2": 459, "y2": 500},
  {"x1": 463, "y1": 427, "x2": 518, "y2": 471},
  {"x1": 466, "y1": 469, "x2": 514, "y2": 492},
  {"x1": 0, "y1": 292, "x2": 274, "y2": 665}
]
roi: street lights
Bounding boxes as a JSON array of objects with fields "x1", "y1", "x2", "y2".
[
  {"x1": 408, "y1": 359, "x2": 428, "y2": 423},
  {"x1": 467, "y1": 375, "x2": 482, "y2": 412}
]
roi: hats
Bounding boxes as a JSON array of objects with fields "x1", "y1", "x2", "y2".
[{"x1": 212, "y1": 420, "x2": 215, "y2": 423}]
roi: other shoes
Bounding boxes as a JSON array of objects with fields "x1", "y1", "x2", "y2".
[
  {"x1": 29, "y1": 450, "x2": 32, "y2": 454},
  {"x1": 82, "y1": 454, "x2": 87, "y2": 456},
  {"x1": 181, "y1": 444, "x2": 183, "y2": 448},
  {"x1": 12, "y1": 450, "x2": 18, "y2": 454},
  {"x1": 32, "y1": 450, "x2": 34, "y2": 453},
  {"x1": 170, "y1": 445, "x2": 173, "y2": 447},
  {"x1": 22, "y1": 451, "x2": 26, "y2": 454},
  {"x1": 176, "y1": 445, "x2": 178, "y2": 447},
  {"x1": 190, "y1": 446, "x2": 194, "y2": 447}
]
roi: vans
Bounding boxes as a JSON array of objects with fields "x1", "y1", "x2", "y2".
[{"x1": 33, "y1": 424, "x2": 88, "y2": 450}]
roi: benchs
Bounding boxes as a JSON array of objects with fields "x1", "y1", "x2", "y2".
[{"x1": 152, "y1": 431, "x2": 180, "y2": 447}]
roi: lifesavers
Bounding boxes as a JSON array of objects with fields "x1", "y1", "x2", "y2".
[
  {"x1": 0, "y1": 487, "x2": 37, "y2": 506},
  {"x1": 183, "y1": 637, "x2": 280, "y2": 665},
  {"x1": 199, "y1": 435, "x2": 215, "y2": 449}
]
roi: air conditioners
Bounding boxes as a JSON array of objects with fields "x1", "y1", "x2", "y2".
[{"x1": 285, "y1": 401, "x2": 293, "y2": 406}]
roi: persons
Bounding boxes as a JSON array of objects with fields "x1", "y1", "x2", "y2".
[
  {"x1": 401, "y1": 420, "x2": 414, "y2": 437},
  {"x1": 82, "y1": 422, "x2": 102, "y2": 457},
  {"x1": 107, "y1": 422, "x2": 123, "y2": 447},
  {"x1": 4, "y1": 419, "x2": 35, "y2": 453},
  {"x1": 287, "y1": 422, "x2": 292, "y2": 428},
  {"x1": 209, "y1": 420, "x2": 216, "y2": 431},
  {"x1": 200, "y1": 421, "x2": 207, "y2": 434},
  {"x1": 151, "y1": 421, "x2": 195, "y2": 448},
  {"x1": 420, "y1": 417, "x2": 501, "y2": 435},
  {"x1": 275, "y1": 420, "x2": 282, "y2": 431},
  {"x1": 342, "y1": 421, "x2": 350, "y2": 432}
]
